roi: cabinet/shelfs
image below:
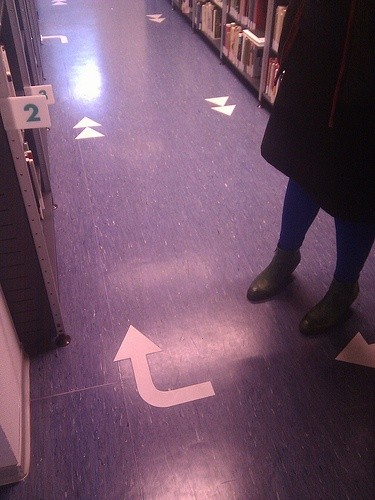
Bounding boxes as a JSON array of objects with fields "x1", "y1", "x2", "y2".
[
  {"x1": 1, "y1": 0, "x2": 72, "y2": 357},
  {"x1": 171, "y1": 0, "x2": 277, "y2": 108}
]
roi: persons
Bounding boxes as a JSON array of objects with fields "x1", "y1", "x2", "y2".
[{"x1": 246, "y1": 1, "x2": 374, "y2": 336}]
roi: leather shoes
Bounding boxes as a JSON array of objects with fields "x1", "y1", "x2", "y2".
[
  {"x1": 300, "y1": 278, "x2": 362, "y2": 336},
  {"x1": 246, "y1": 248, "x2": 302, "y2": 302}
]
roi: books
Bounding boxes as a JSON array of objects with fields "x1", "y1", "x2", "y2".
[
  {"x1": 0, "y1": 43, "x2": 45, "y2": 220},
  {"x1": 171, "y1": 1, "x2": 289, "y2": 103}
]
